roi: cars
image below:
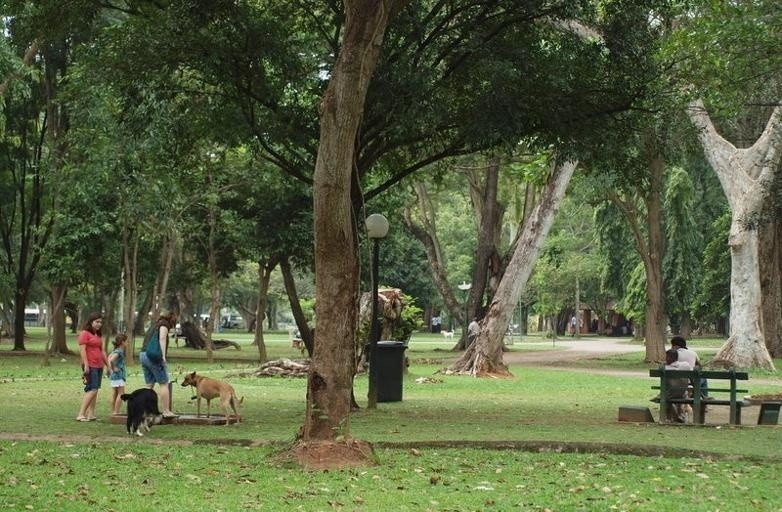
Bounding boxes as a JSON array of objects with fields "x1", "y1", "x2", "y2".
[{"x1": 175, "y1": 312, "x2": 244, "y2": 336}]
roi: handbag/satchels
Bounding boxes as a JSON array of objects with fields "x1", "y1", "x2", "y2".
[{"x1": 146, "y1": 319, "x2": 162, "y2": 364}]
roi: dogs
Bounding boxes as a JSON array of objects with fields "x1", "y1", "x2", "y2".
[
  {"x1": 181, "y1": 371, "x2": 245, "y2": 428},
  {"x1": 440, "y1": 329, "x2": 455, "y2": 340},
  {"x1": 119, "y1": 388, "x2": 165, "y2": 437}
]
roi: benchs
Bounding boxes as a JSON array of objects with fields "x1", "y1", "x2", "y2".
[{"x1": 648, "y1": 368, "x2": 751, "y2": 425}]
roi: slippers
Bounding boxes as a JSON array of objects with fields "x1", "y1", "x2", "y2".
[{"x1": 76, "y1": 417, "x2": 90, "y2": 421}]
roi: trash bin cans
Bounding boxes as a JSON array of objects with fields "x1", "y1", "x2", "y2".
[{"x1": 363, "y1": 339, "x2": 408, "y2": 403}]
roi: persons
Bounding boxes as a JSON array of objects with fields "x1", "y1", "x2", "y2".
[
  {"x1": 437, "y1": 315, "x2": 442, "y2": 333},
  {"x1": 431, "y1": 316, "x2": 437, "y2": 333},
  {"x1": 175, "y1": 322, "x2": 181, "y2": 338},
  {"x1": 107, "y1": 333, "x2": 129, "y2": 415},
  {"x1": 669, "y1": 336, "x2": 709, "y2": 413},
  {"x1": 75, "y1": 311, "x2": 107, "y2": 422},
  {"x1": 139, "y1": 311, "x2": 180, "y2": 418},
  {"x1": 571, "y1": 313, "x2": 576, "y2": 337},
  {"x1": 657, "y1": 348, "x2": 716, "y2": 423}
]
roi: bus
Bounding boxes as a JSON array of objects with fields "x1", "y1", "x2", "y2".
[{"x1": 22, "y1": 309, "x2": 47, "y2": 326}]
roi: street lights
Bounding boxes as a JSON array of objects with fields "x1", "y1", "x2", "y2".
[
  {"x1": 456, "y1": 281, "x2": 473, "y2": 348},
  {"x1": 364, "y1": 214, "x2": 389, "y2": 406}
]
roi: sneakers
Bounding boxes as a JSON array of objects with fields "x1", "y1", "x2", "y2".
[{"x1": 163, "y1": 411, "x2": 179, "y2": 419}]
